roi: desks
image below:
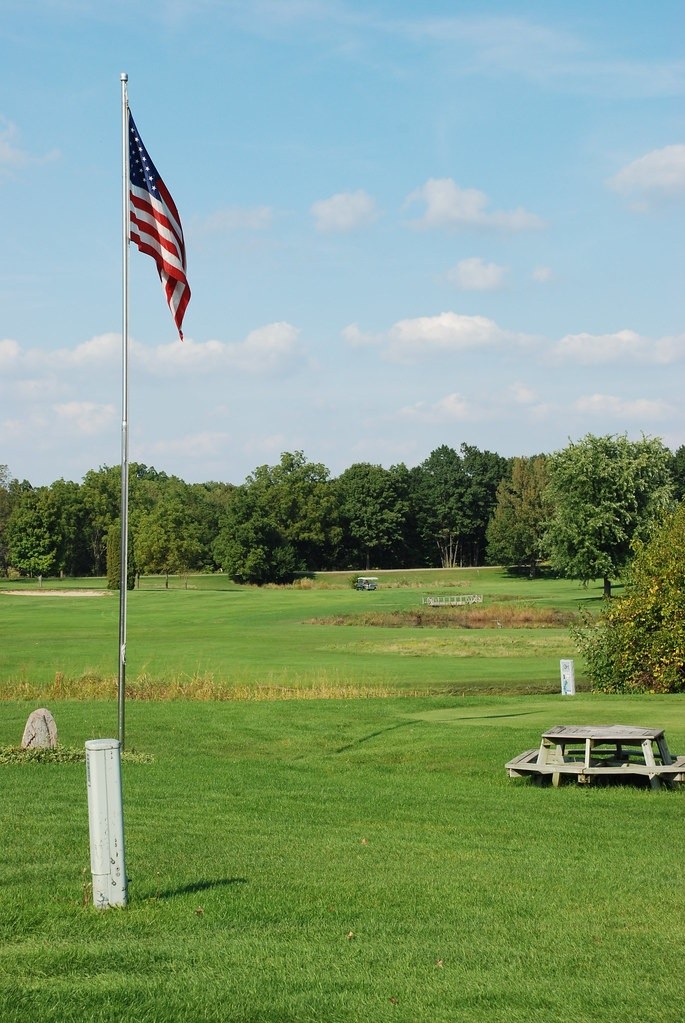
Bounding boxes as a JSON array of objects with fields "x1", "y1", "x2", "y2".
[{"x1": 533, "y1": 724, "x2": 674, "y2": 791}]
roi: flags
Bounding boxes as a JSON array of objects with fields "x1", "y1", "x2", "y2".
[{"x1": 127, "y1": 110, "x2": 191, "y2": 342}]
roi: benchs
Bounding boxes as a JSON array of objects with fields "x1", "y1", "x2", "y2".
[{"x1": 505, "y1": 747, "x2": 685, "y2": 791}]
